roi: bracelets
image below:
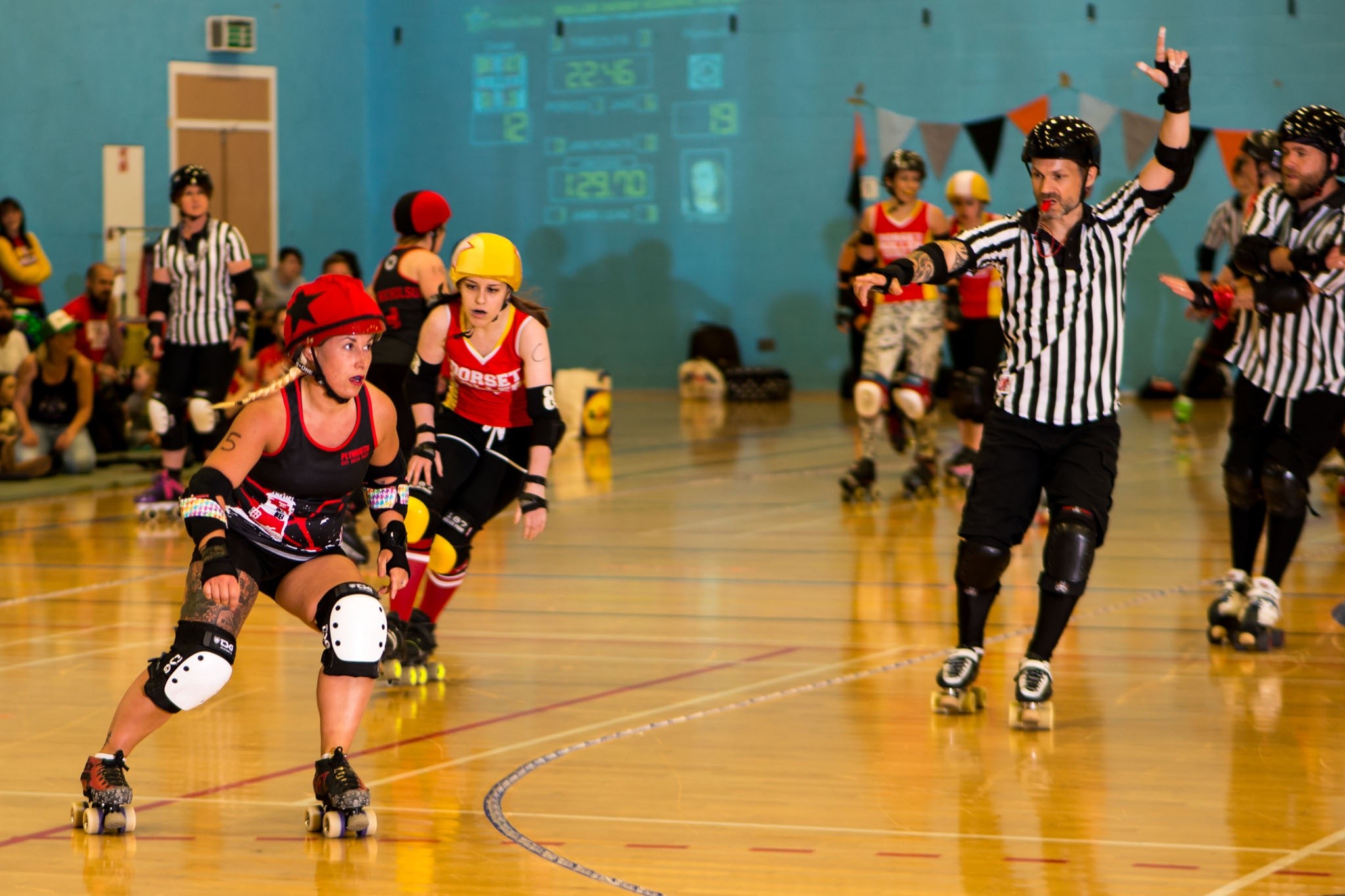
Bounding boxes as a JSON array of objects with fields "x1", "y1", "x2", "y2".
[
  {"x1": 415, "y1": 423, "x2": 436, "y2": 434},
  {"x1": 524, "y1": 473, "x2": 545, "y2": 486}
]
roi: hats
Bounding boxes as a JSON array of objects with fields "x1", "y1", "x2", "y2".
[{"x1": 41, "y1": 307, "x2": 83, "y2": 337}]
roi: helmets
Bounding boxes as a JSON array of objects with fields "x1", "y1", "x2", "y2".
[
  {"x1": 392, "y1": 190, "x2": 452, "y2": 235},
  {"x1": 447, "y1": 232, "x2": 522, "y2": 293},
  {"x1": 1275, "y1": 104, "x2": 1345, "y2": 157},
  {"x1": 169, "y1": 164, "x2": 213, "y2": 205},
  {"x1": 284, "y1": 273, "x2": 385, "y2": 360},
  {"x1": 1240, "y1": 128, "x2": 1278, "y2": 168},
  {"x1": 880, "y1": 150, "x2": 925, "y2": 186},
  {"x1": 1021, "y1": 115, "x2": 1101, "y2": 175},
  {"x1": 946, "y1": 171, "x2": 992, "y2": 204}
]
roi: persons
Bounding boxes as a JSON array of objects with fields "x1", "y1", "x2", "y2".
[
  {"x1": 380, "y1": 231, "x2": 568, "y2": 665},
  {"x1": 836, "y1": 148, "x2": 950, "y2": 489},
  {"x1": 0, "y1": 197, "x2": 157, "y2": 476},
  {"x1": 1158, "y1": 103, "x2": 1345, "y2": 631},
  {"x1": 365, "y1": 188, "x2": 450, "y2": 538},
  {"x1": 217, "y1": 245, "x2": 359, "y2": 424},
  {"x1": 852, "y1": 24, "x2": 1191, "y2": 704},
  {"x1": 131, "y1": 165, "x2": 258, "y2": 507},
  {"x1": 81, "y1": 270, "x2": 411, "y2": 809},
  {"x1": 832, "y1": 230, "x2": 908, "y2": 452},
  {"x1": 942, "y1": 169, "x2": 1006, "y2": 479}
]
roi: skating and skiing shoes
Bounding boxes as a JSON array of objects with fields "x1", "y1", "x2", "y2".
[
  {"x1": 931, "y1": 645, "x2": 985, "y2": 714},
  {"x1": 1206, "y1": 567, "x2": 1254, "y2": 645},
  {"x1": 943, "y1": 442, "x2": 978, "y2": 487},
  {"x1": 900, "y1": 450, "x2": 939, "y2": 498},
  {"x1": 1233, "y1": 576, "x2": 1286, "y2": 650},
  {"x1": 1172, "y1": 393, "x2": 1193, "y2": 425},
  {"x1": 306, "y1": 747, "x2": 377, "y2": 839},
  {"x1": 394, "y1": 608, "x2": 447, "y2": 686},
  {"x1": 1007, "y1": 657, "x2": 1055, "y2": 730},
  {"x1": 70, "y1": 749, "x2": 135, "y2": 834},
  {"x1": 839, "y1": 456, "x2": 883, "y2": 501},
  {"x1": 132, "y1": 471, "x2": 183, "y2": 521},
  {"x1": 373, "y1": 609, "x2": 408, "y2": 681}
]
